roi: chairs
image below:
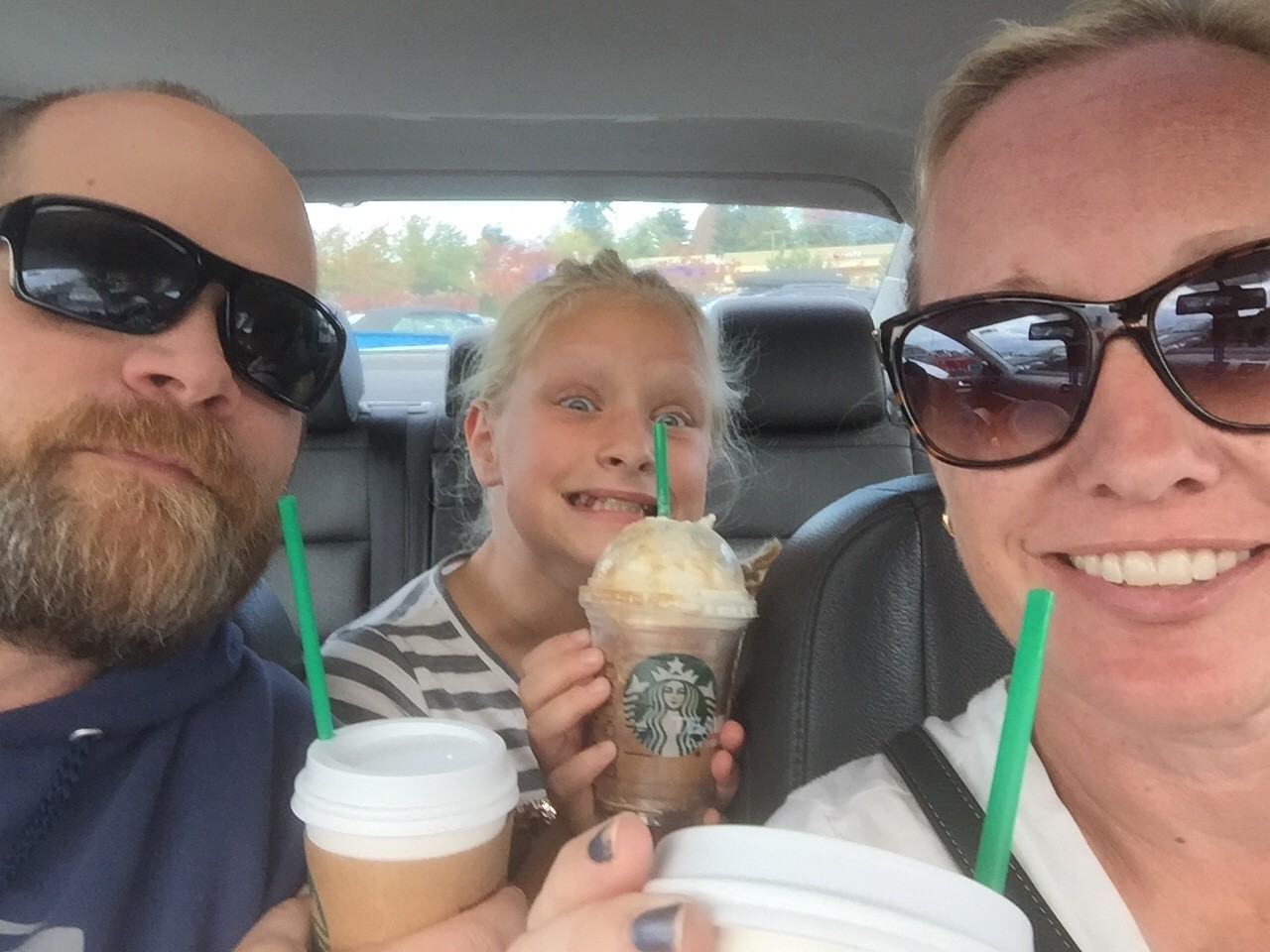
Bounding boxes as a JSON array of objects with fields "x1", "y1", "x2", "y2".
[
  {"x1": 707, "y1": 291, "x2": 916, "y2": 559},
  {"x1": 261, "y1": 296, "x2": 406, "y2": 639},
  {"x1": 741, "y1": 474, "x2": 1011, "y2": 833},
  {"x1": 407, "y1": 326, "x2": 494, "y2": 576}
]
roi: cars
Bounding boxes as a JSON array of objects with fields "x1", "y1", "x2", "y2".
[{"x1": 350, "y1": 306, "x2": 484, "y2": 346}]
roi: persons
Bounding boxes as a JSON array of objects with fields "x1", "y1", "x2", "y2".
[
  {"x1": 306, "y1": 242, "x2": 750, "y2": 916},
  {"x1": 504, "y1": 1, "x2": 1270, "y2": 952},
  {"x1": 1, "y1": 79, "x2": 527, "y2": 952}
]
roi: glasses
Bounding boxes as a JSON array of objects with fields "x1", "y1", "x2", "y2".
[
  {"x1": 0, "y1": 194, "x2": 347, "y2": 415},
  {"x1": 879, "y1": 236, "x2": 1270, "y2": 469}
]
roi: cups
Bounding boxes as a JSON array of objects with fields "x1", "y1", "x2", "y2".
[
  {"x1": 642, "y1": 824, "x2": 1034, "y2": 952},
  {"x1": 579, "y1": 517, "x2": 760, "y2": 825},
  {"x1": 290, "y1": 717, "x2": 520, "y2": 952}
]
